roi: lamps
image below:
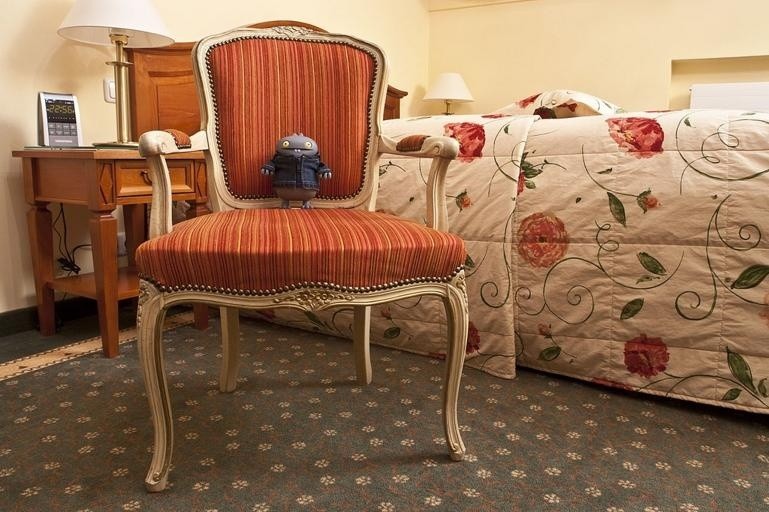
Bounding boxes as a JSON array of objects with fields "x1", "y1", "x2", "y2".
[
  {"x1": 56, "y1": 0, "x2": 178, "y2": 150},
  {"x1": 421, "y1": 72, "x2": 477, "y2": 116}
]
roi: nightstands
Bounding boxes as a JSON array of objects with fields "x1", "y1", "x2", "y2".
[{"x1": 12, "y1": 144, "x2": 220, "y2": 359}]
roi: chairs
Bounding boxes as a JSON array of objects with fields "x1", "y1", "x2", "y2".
[{"x1": 111, "y1": 15, "x2": 476, "y2": 498}]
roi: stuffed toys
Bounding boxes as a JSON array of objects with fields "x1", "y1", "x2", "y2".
[{"x1": 261, "y1": 133, "x2": 333, "y2": 210}]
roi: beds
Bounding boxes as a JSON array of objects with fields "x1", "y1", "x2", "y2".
[{"x1": 129, "y1": 19, "x2": 768, "y2": 417}]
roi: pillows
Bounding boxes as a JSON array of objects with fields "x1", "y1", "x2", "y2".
[{"x1": 479, "y1": 87, "x2": 624, "y2": 121}]
